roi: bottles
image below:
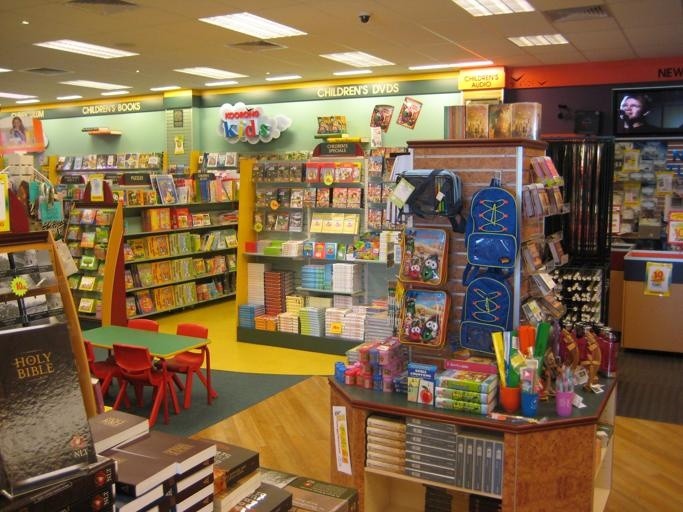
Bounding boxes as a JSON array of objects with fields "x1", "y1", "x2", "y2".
[{"x1": 573, "y1": 320, "x2": 622, "y2": 379}]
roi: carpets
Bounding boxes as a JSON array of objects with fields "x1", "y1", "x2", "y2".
[{"x1": 95, "y1": 347, "x2": 313, "y2": 438}]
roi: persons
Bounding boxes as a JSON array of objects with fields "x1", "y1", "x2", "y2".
[
  {"x1": 329, "y1": 116, "x2": 347, "y2": 133},
  {"x1": 619, "y1": 93, "x2": 655, "y2": 130},
  {"x1": 316, "y1": 116, "x2": 329, "y2": 134},
  {"x1": 9, "y1": 116, "x2": 27, "y2": 145}
]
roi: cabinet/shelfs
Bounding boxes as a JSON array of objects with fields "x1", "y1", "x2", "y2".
[
  {"x1": 46, "y1": 151, "x2": 409, "y2": 355},
  {"x1": 328, "y1": 375, "x2": 618, "y2": 512}
]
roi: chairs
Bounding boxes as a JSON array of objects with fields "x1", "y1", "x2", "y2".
[
  {"x1": 154, "y1": 324, "x2": 217, "y2": 408},
  {"x1": 113, "y1": 344, "x2": 179, "y2": 428},
  {"x1": 83, "y1": 341, "x2": 130, "y2": 409}
]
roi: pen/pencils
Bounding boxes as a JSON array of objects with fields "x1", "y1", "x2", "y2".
[{"x1": 556, "y1": 365, "x2": 574, "y2": 393}]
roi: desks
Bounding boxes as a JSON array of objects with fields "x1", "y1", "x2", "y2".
[{"x1": 81, "y1": 325, "x2": 212, "y2": 425}]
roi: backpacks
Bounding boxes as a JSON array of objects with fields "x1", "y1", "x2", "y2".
[{"x1": 395, "y1": 169, "x2": 521, "y2": 358}]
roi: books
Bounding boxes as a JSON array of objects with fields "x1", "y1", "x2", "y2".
[
  {"x1": 1, "y1": 323, "x2": 359, "y2": 511},
  {"x1": 81, "y1": 127, "x2": 107, "y2": 132},
  {"x1": 442, "y1": 102, "x2": 542, "y2": 139},
  {"x1": 518, "y1": 156, "x2": 566, "y2": 325},
  {"x1": 1, "y1": 151, "x2": 241, "y2": 317},
  {"x1": 313, "y1": 134, "x2": 369, "y2": 144},
  {"x1": 366, "y1": 353, "x2": 505, "y2": 496},
  {"x1": 0, "y1": 112, "x2": 34, "y2": 147},
  {"x1": 86, "y1": 130, "x2": 122, "y2": 136},
  {"x1": 239, "y1": 148, "x2": 408, "y2": 342}
]
